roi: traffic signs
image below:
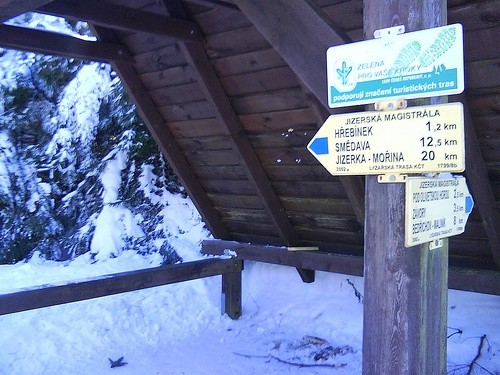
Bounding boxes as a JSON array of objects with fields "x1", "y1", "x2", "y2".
[
  {"x1": 324, "y1": 23, "x2": 465, "y2": 110},
  {"x1": 403, "y1": 175, "x2": 475, "y2": 250},
  {"x1": 307, "y1": 102, "x2": 467, "y2": 176}
]
